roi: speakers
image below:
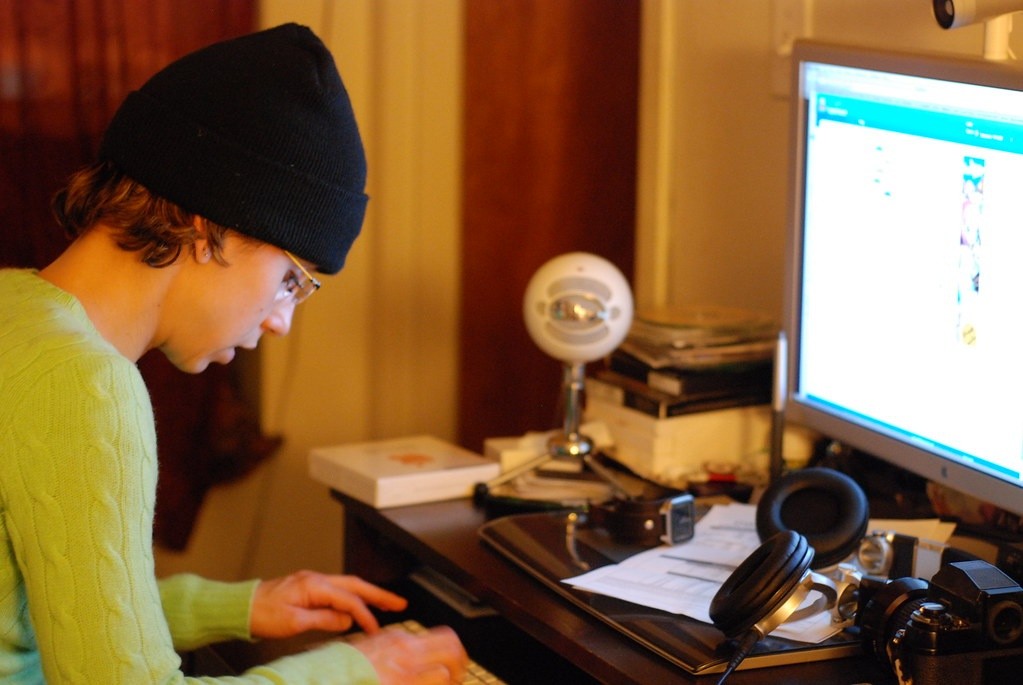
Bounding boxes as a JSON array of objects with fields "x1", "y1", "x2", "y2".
[{"x1": 522, "y1": 249, "x2": 634, "y2": 365}]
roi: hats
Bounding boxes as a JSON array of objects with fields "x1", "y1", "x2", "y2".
[{"x1": 99, "y1": 21, "x2": 370, "y2": 275}]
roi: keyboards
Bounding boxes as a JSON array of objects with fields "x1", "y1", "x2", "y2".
[{"x1": 299, "y1": 619, "x2": 509, "y2": 684}]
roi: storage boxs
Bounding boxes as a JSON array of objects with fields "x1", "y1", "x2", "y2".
[{"x1": 583, "y1": 399, "x2": 812, "y2": 486}]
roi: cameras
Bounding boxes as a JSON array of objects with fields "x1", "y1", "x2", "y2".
[{"x1": 874, "y1": 560, "x2": 1023, "y2": 685}]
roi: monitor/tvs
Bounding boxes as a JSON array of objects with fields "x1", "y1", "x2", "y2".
[{"x1": 784, "y1": 40, "x2": 1023, "y2": 559}]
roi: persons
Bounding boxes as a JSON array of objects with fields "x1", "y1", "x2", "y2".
[{"x1": 0, "y1": 23, "x2": 469, "y2": 685}]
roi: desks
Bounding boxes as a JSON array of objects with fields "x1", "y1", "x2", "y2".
[{"x1": 330, "y1": 457, "x2": 873, "y2": 685}]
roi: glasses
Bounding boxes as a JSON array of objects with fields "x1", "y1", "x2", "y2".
[{"x1": 286, "y1": 249, "x2": 321, "y2": 307}]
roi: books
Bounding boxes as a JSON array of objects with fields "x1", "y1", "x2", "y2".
[
  {"x1": 308, "y1": 435, "x2": 501, "y2": 507},
  {"x1": 584, "y1": 313, "x2": 819, "y2": 478}
]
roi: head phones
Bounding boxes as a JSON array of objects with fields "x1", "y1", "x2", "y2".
[{"x1": 710, "y1": 465, "x2": 870, "y2": 642}]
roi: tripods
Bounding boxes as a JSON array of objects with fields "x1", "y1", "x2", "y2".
[{"x1": 473, "y1": 362, "x2": 645, "y2": 508}]
